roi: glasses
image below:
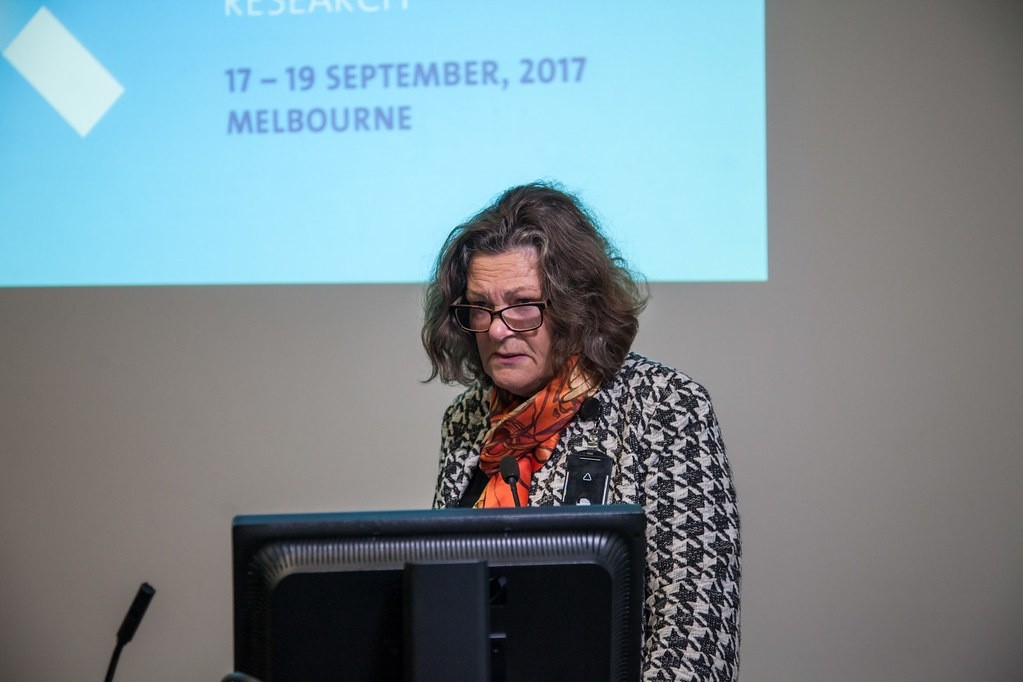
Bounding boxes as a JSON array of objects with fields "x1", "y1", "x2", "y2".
[{"x1": 449, "y1": 295, "x2": 551, "y2": 334}]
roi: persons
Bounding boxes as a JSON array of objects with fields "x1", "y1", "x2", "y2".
[{"x1": 420, "y1": 182, "x2": 740, "y2": 682}]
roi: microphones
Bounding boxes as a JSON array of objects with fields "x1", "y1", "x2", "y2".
[
  {"x1": 105, "y1": 582, "x2": 155, "y2": 682},
  {"x1": 498, "y1": 455, "x2": 523, "y2": 508}
]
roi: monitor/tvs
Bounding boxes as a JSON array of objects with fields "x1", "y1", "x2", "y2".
[{"x1": 232, "y1": 504, "x2": 644, "y2": 682}]
